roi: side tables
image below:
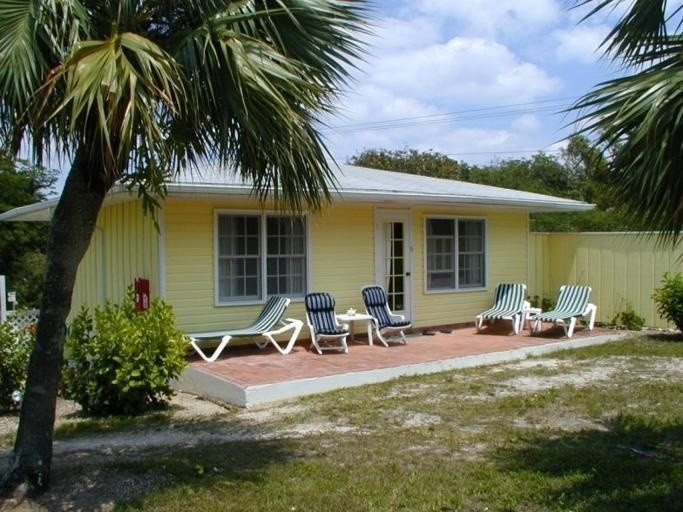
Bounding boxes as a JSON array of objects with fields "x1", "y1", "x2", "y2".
[{"x1": 337, "y1": 312, "x2": 374, "y2": 346}]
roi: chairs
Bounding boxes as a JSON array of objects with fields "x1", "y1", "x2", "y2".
[
  {"x1": 302, "y1": 292, "x2": 350, "y2": 355},
  {"x1": 180, "y1": 294, "x2": 303, "y2": 362},
  {"x1": 361, "y1": 283, "x2": 410, "y2": 349},
  {"x1": 534, "y1": 284, "x2": 597, "y2": 337},
  {"x1": 473, "y1": 283, "x2": 531, "y2": 335}
]
